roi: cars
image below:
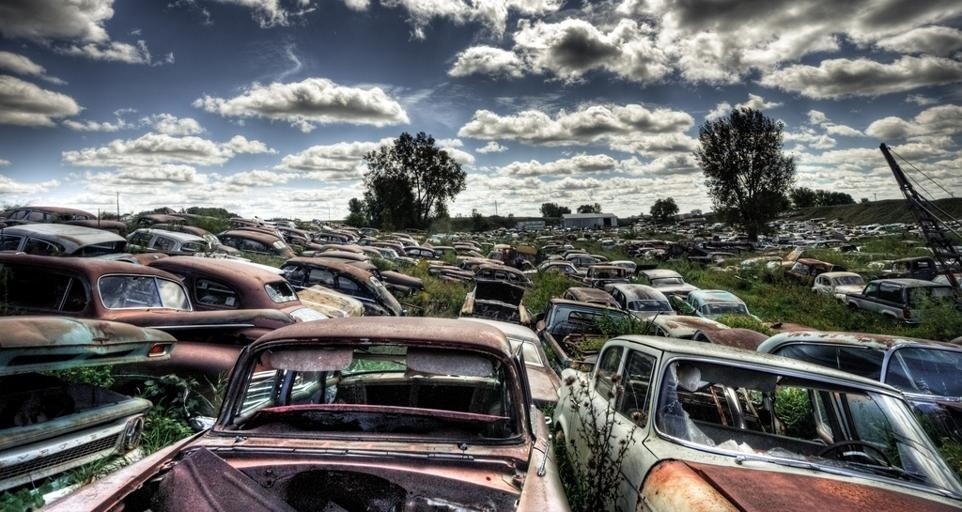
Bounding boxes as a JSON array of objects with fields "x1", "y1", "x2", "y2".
[
  {"x1": 1, "y1": 315, "x2": 178, "y2": 500},
  {"x1": 1, "y1": 207, "x2": 961, "y2": 426},
  {"x1": 552, "y1": 335, "x2": 961, "y2": 511},
  {"x1": 37, "y1": 316, "x2": 572, "y2": 511},
  {"x1": 691, "y1": 328, "x2": 961, "y2": 459}
]
